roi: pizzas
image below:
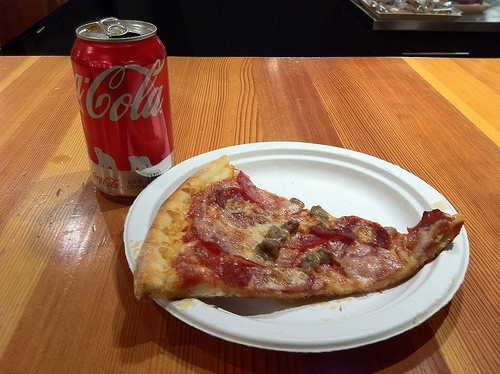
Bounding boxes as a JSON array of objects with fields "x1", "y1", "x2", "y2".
[{"x1": 133, "y1": 155, "x2": 464, "y2": 298}]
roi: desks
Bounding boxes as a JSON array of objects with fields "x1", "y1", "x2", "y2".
[{"x1": 0, "y1": 55, "x2": 500, "y2": 374}]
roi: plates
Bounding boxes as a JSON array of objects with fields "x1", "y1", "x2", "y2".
[
  {"x1": 453, "y1": 1, "x2": 492, "y2": 13},
  {"x1": 122, "y1": 141, "x2": 470, "y2": 352}
]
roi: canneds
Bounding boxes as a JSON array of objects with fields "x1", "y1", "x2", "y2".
[{"x1": 70, "y1": 17, "x2": 173, "y2": 198}]
